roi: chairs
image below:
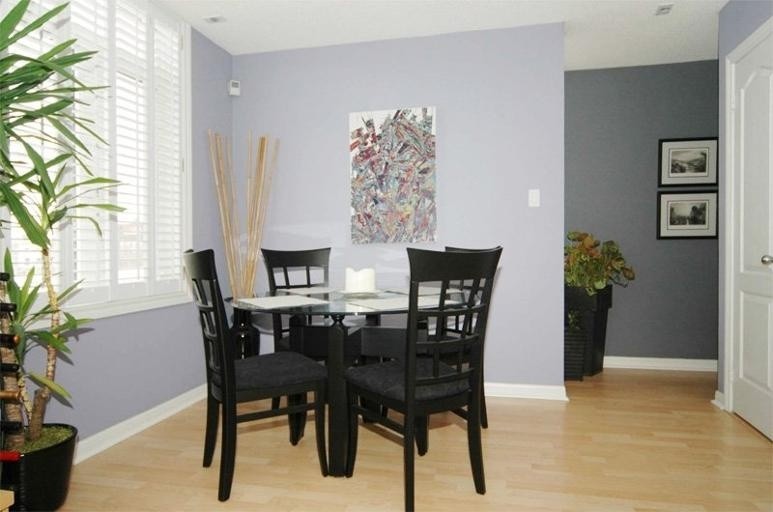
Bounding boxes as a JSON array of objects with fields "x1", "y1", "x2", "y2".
[
  {"x1": 232, "y1": 244, "x2": 503, "y2": 478},
  {"x1": 344, "y1": 247, "x2": 502, "y2": 512},
  {"x1": 183, "y1": 248, "x2": 328, "y2": 502}
]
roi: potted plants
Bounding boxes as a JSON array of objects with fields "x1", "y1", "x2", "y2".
[
  {"x1": 0, "y1": 0, "x2": 131, "y2": 512},
  {"x1": 565, "y1": 229, "x2": 639, "y2": 386}
]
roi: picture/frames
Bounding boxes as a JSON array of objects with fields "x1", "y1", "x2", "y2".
[{"x1": 654, "y1": 136, "x2": 716, "y2": 241}]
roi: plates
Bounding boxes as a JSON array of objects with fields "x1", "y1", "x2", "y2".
[{"x1": 340, "y1": 290, "x2": 380, "y2": 298}]
